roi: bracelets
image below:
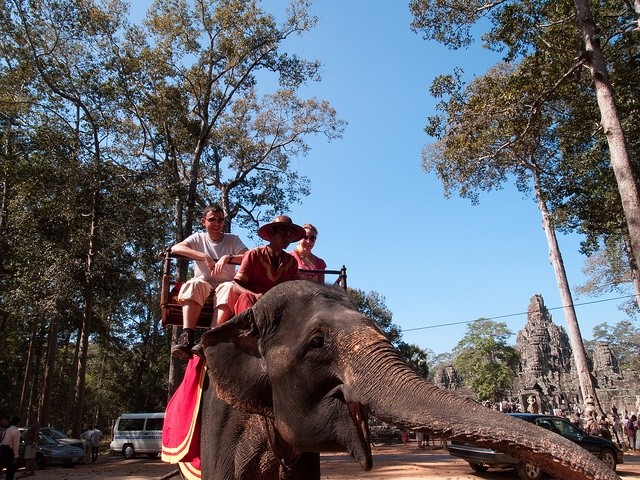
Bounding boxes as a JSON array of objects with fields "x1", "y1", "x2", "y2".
[{"x1": 228, "y1": 254, "x2": 233, "y2": 263}]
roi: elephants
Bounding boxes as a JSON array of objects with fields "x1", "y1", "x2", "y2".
[{"x1": 197, "y1": 278, "x2": 625, "y2": 480}]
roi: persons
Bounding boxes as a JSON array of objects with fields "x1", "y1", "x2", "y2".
[
  {"x1": 423, "y1": 433, "x2": 430, "y2": 446},
  {"x1": 0, "y1": 417, "x2": 20, "y2": 480},
  {"x1": 401, "y1": 430, "x2": 408, "y2": 446},
  {"x1": 88, "y1": 424, "x2": 103, "y2": 464},
  {"x1": 20, "y1": 414, "x2": 40, "y2": 478},
  {"x1": 415, "y1": 432, "x2": 423, "y2": 447},
  {"x1": 80, "y1": 425, "x2": 92, "y2": 465},
  {"x1": 233, "y1": 216, "x2": 306, "y2": 316},
  {"x1": 485, "y1": 401, "x2": 541, "y2": 414},
  {"x1": 572, "y1": 413, "x2": 583, "y2": 431},
  {"x1": 585, "y1": 406, "x2": 640, "y2": 452},
  {"x1": 288, "y1": 224, "x2": 327, "y2": 284},
  {"x1": 170, "y1": 202, "x2": 249, "y2": 361}
]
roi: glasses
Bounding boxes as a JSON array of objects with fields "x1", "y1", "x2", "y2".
[
  {"x1": 205, "y1": 218, "x2": 224, "y2": 221},
  {"x1": 304, "y1": 236, "x2": 317, "y2": 240}
]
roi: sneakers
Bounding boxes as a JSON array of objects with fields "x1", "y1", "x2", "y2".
[
  {"x1": 190, "y1": 339, "x2": 204, "y2": 360},
  {"x1": 172, "y1": 332, "x2": 194, "y2": 360}
]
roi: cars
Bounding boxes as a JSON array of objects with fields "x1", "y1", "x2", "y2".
[
  {"x1": 445, "y1": 411, "x2": 625, "y2": 479},
  {"x1": 38, "y1": 427, "x2": 84, "y2": 454},
  {"x1": 6, "y1": 427, "x2": 83, "y2": 469}
]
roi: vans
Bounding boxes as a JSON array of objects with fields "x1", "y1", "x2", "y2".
[{"x1": 109, "y1": 412, "x2": 165, "y2": 459}]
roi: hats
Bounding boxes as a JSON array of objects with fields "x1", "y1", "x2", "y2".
[{"x1": 258, "y1": 216, "x2": 306, "y2": 242}]
